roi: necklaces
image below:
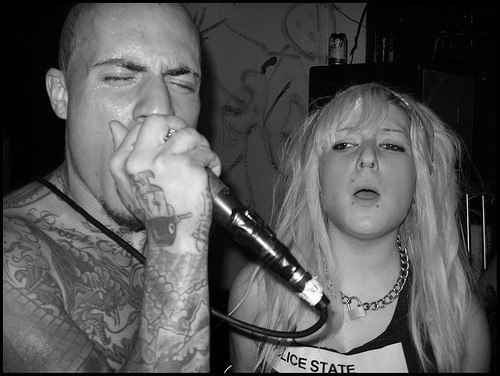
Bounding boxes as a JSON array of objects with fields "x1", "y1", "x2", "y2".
[{"x1": 318, "y1": 229, "x2": 411, "y2": 322}]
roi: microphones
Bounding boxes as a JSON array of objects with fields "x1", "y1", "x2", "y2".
[{"x1": 163, "y1": 129, "x2": 331, "y2": 312}]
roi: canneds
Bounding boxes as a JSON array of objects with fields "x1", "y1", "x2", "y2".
[{"x1": 328, "y1": 32, "x2": 348, "y2": 66}]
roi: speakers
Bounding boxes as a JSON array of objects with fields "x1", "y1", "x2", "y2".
[{"x1": 308, "y1": 65, "x2": 476, "y2": 163}]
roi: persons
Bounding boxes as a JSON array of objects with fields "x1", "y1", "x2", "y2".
[
  {"x1": 227, "y1": 81, "x2": 494, "y2": 376},
  {"x1": 1, "y1": 1, "x2": 222, "y2": 374}
]
